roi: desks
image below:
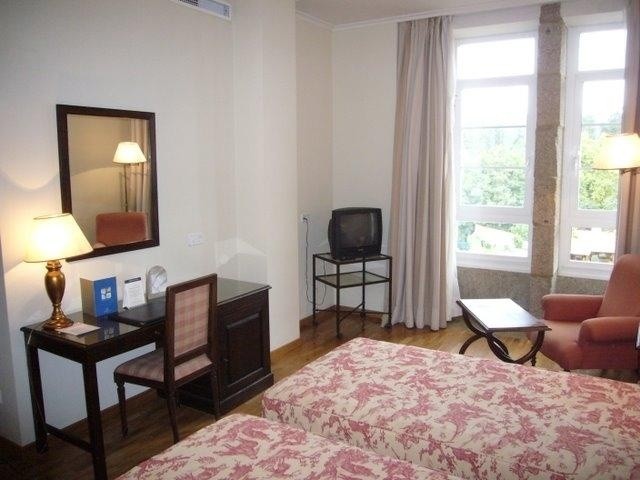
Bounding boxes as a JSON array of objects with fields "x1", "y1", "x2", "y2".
[
  {"x1": 20, "y1": 277, "x2": 274, "y2": 479},
  {"x1": 312, "y1": 253, "x2": 392, "y2": 339},
  {"x1": 456, "y1": 298, "x2": 553, "y2": 367}
]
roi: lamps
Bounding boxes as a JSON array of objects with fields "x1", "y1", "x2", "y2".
[
  {"x1": 591, "y1": 133, "x2": 640, "y2": 254},
  {"x1": 113, "y1": 142, "x2": 147, "y2": 212},
  {"x1": 24, "y1": 213, "x2": 94, "y2": 328}
]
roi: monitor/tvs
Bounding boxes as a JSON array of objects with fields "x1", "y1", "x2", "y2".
[{"x1": 329, "y1": 207, "x2": 382, "y2": 261}]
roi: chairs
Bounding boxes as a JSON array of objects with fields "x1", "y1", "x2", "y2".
[
  {"x1": 525, "y1": 253, "x2": 640, "y2": 378},
  {"x1": 114, "y1": 273, "x2": 221, "y2": 444},
  {"x1": 94, "y1": 211, "x2": 149, "y2": 249}
]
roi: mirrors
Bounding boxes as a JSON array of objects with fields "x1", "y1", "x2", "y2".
[{"x1": 56, "y1": 105, "x2": 159, "y2": 263}]
